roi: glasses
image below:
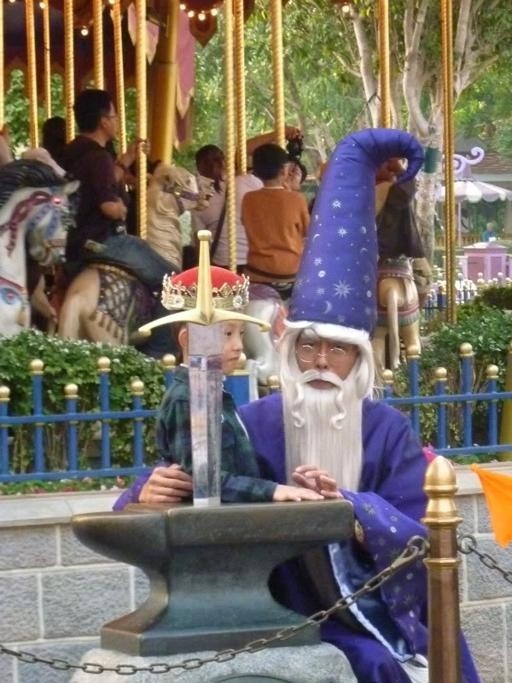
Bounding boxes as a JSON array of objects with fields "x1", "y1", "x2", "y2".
[{"x1": 293, "y1": 344, "x2": 359, "y2": 367}]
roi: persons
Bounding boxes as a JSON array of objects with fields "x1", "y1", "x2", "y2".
[
  {"x1": 1, "y1": 91, "x2": 429, "y2": 358},
  {"x1": 482, "y1": 222, "x2": 498, "y2": 242},
  {"x1": 159, "y1": 266, "x2": 324, "y2": 502},
  {"x1": 109, "y1": 322, "x2": 480, "y2": 683}
]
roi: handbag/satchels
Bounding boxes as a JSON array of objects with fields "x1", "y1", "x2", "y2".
[{"x1": 182, "y1": 245, "x2": 201, "y2": 270}]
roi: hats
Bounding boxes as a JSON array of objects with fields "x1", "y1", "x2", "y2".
[
  {"x1": 284, "y1": 129, "x2": 423, "y2": 341},
  {"x1": 161, "y1": 264, "x2": 250, "y2": 310}
]
